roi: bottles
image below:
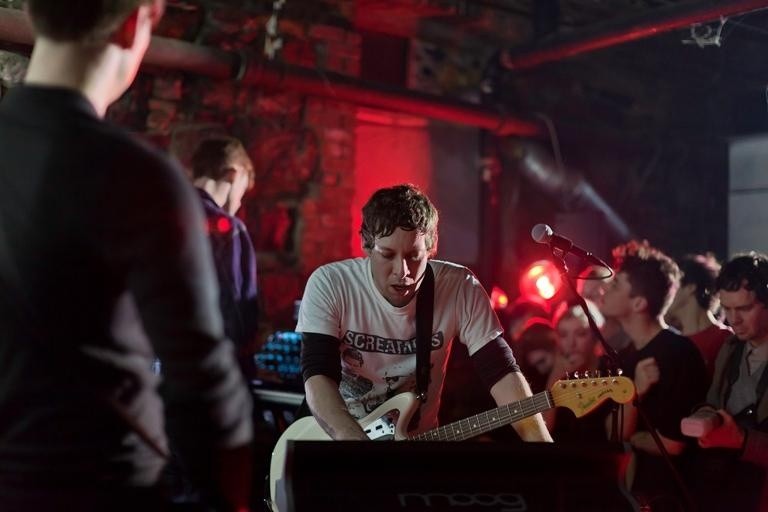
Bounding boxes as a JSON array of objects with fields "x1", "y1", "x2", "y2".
[{"x1": 680, "y1": 408, "x2": 722, "y2": 438}]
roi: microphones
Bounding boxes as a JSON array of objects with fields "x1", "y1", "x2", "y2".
[{"x1": 531, "y1": 223, "x2": 605, "y2": 267}]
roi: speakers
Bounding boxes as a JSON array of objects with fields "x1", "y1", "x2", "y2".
[{"x1": 286, "y1": 442, "x2": 635, "y2": 512}]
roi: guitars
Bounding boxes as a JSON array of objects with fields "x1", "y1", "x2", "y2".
[{"x1": 269, "y1": 369, "x2": 636, "y2": 512}]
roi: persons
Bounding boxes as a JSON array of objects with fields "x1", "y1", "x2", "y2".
[
  {"x1": 183, "y1": 134, "x2": 257, "y2": 359},
  {"x1": 460, "y1": 242, "x2": 767, "y2": 512},
  {"x1": 295, "y1": 184, "x2": 554, "y2": 443},
  {"x1": 0, "y1": 0, "x2": 257, "y2": 511}
]
